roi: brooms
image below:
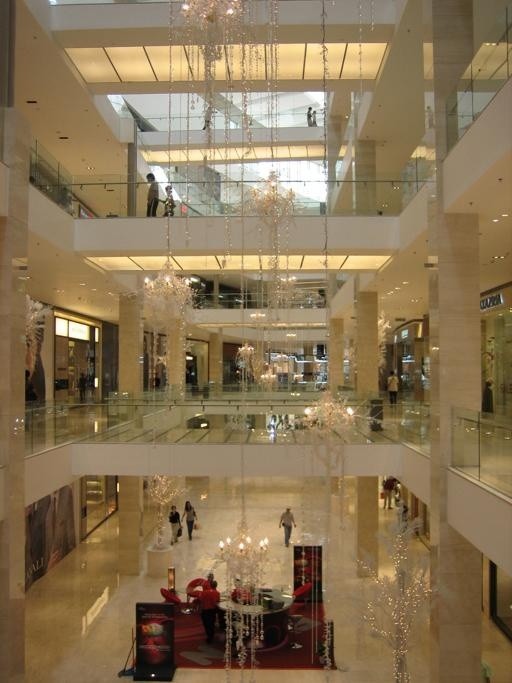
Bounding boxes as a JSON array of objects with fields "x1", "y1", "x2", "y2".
[{"x1": 118, "y1": 637, "x2": 136, "y2": 677}]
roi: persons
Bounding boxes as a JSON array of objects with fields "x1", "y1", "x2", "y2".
[
  {"x1": 155, "y1": 372, "x2": 162, "y2": 388},
  {"x1": 276, "y1": 506, "x2": 298, "y2": 551},
  {"x1": 310, "y1": 111, "x2": 317, "y2": 125},
  {"x1": 26, "y1": 369, "x2": 40, "y2": 430},
  {"x1": 305, "y1": 105, "x2": 313, "y2": 129},
  {"x1": 144, "y1": 171, "x2": 159, "y2": 217},
  {"x1": 385, "y1": 370, "x2": 399, "y2": 406},
  {"x1": 77, "y1": 372, "x2": 86, "y2": 401},
  {"x1": 380, "y1": 474, "x2": 396, "y2": 511},
  {"x1": 179, "y1": 498, "x2": 198, "y2": 542},
  {"x1": 200, "y1": 104, "x2": 220, "y2": 129},
  {"x1": 167, "y1": 504, "x2": 185, "y2": 545},
  {"x1": 157, "y1": 184, "x2": 177, "y2": 217},
  {"x1": 201, "y1": 571, "x2": 215, "y2": 592},
  {"x1": 199, "y1": 579, "x2": 221, "y2": 646},
  {"x1": 482, "y1": 370, "x2": 494, "y2": 410}
]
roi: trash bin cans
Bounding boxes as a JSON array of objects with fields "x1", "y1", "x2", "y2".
[{"x1": 370, "y1": 400, "x2": 383, "y2": 432}]
